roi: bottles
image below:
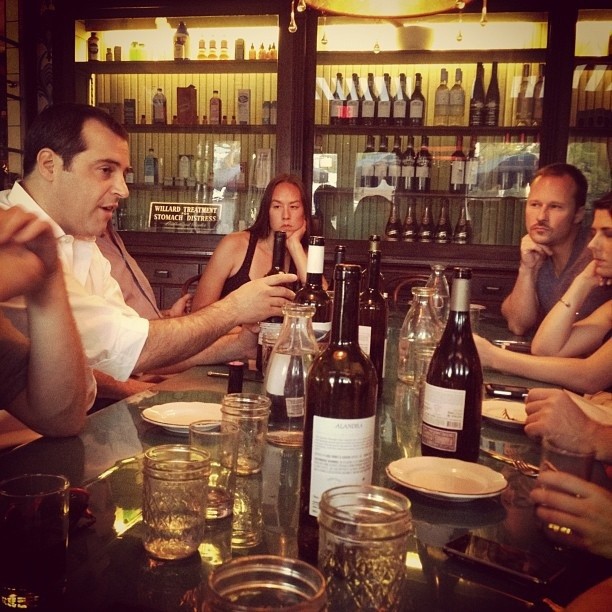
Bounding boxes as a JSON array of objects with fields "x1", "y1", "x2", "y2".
[
  {"x1": 375, "y1": 134, "x2": 389, "y2": 187},
  {"x1": 454, "y1": 199, "x2": 473, "y2": 245},
  {"x1": 402, "y1": 198, "x2": 418, "y2": 242},
  {"x1": 106, "y1": 47, "x2": 113, "y2": 61},
  {"x1": 193, "y1": 143, "x2": 203, "y2": 186},
  {"x1": 173, "y1": 37, "x2": 186, "y2": 61},
  {"x1": 385, "y1": 195, "x2": 402, "y2": 241},
  {"x1": 361, "y1": 134, "x2": 376, "y2": 187},
  {"x1": 209, "y1": 90, "x2": 222, "y2": 125},
  {"x1": 450, "y1": 134, "x2": 467, "y2": 193},
  {"x1": 158, "y1": 157, "x2": 165, "y2": 184},
  {"x1": 329, "y1": 73, "x2": 347, "y2": 127},
  {"x1": 297, "y1": 263, "x2": 380, "y2": 560},
  {"x1": 361, "y1": 72, "x2": 378, "y2": 127},
  {"x1": 256, "y1": 154, "x2": 271, "y2": 189},
  {"x1": 200, "y1": 145, "x2": 211, "y2": 186},
  {"x1": 293, "y1": 235, "x2": 332, "y2": 353},
  {"x1": 464, "y1": 133, "x2": 482, "y2": 193},
  {"x1": 249, "y1": 43, "x2": 257, "y2": 60},
  {"x1": 515, "y1": 64, "x2": 533, "y2": 127},
  {"x1": 259, "y1": 42, "x2": 267, "y2": 59},
  {"x1": 325, "y1": 290, "x2": 335, "y2": 303},
  {"x1": 346, "y1": 73, "x2": 362, "y2": 127},
  {"x1": 118, "y1": 198, "x2": 129, "y2": 232},
  {"x1": 312, "y1": 202, "x2": 323, "y2": 237},
  {"x1": 358, "y1": 234, "x2": 385, "y2": 394},
  {"x1": 448, "y1": 68, "x2": 466, "y2": 126},
  {"x1": 533, "y1": 63, "x2": 546, "y2": 126},
  {"x1": 125, "y1": 166, "x2": 135, "y2": 184},
  {"x1": 396, "y1": 379, "x2": 424, "y2": 458},
  {"x1": 267, "y1": 45, "x2": 272, "y2": 59},
  {"x1": 377, "y1": 72, "x2": 393, "y2": 127},
  {"x1": 313, "y1": 130, "x2": 329, "y2": 184},
  {"x1": 270, "y1": 42, "x2": 278, "y2": 60},
  {"x1": 424, "y1": 261, "x2": 450, "y2": 312},
  {"x1": 392, "y1": 73, "x2": 410, "y2": 127},
  {"x1": 419, "y1": 198, "x2": 434, "y2": 242},
  {"x1": 277, "y1": 450, "x2": 306, "y2": 553},
  {"x1": 415, "y1": 135, "x2": 432, "y2": 192},
  {"x1": 402, "y1": 134, "x2": 416, "y2": 192},
  {"x1": 144, "y1": 148, "x2": 158, "y2": 186},
  {"x1": 256, "y1": 230, "x2": 287, "y2": 382},
  {"x1": 197, "y1": 39, "x2": 207, "y2": 60},
  {"x1": 388, "y1": 134, "x2": 403, "y2": 190},
  {"x1": 470, "y1": 61, "x2": 486, "y2": 127},
  {"x1": 208, "y1": 39, "x2": 219, "y2": 60},
  {"x1": 515, "y1": 132, "x2": 536, "y2": 196},
  {"x1": 219, "y1": 39, "x2": 230, "y2": 60},
  {"x1": 397, "y1": 286, "x2": 444, "y2": 385},
  {"x1": 249, "y1": 152, "x2": 258, "y2": 185},
  {"x1": 87, "y1": 32, "x2": 100, "y2": 61},
  {"x1": 420, "y1": 267, "x2": 484, "y2": 462},
  {"x1": 173, "y1": 20, "x2": 192, "y2": 60},
  {"x1": 259, "y1": 304, "x2": 321, "y2": 448},
  {"x1": 326, "y1": 244, "x2": 347, "y2": 291},
  {"x1": 433, "y1": 68, "x2": 450, "y2": 126},
  {"x1": 360, "y1": 249, "x2": 382, "y2": 292},
  {"x1": 434, "y1": 198, "x2": 454, "y2": 244},
  {"x1": 499, "y1": 130, "x2": 515, "y2": 190},
  {"x1": 485, "y1": 60, "x2": 500, "y2": 126},
  {"x1": 409, "y1": 73, "x2": 426, "y2": 127}
]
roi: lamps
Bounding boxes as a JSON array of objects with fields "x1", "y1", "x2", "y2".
[{"x1": 285, "y1": 0, "x2": 489, "y2": 54}]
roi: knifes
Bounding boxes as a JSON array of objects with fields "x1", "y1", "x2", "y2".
[{"x1": 480, "y1": 446, "x2": 540, "y2": 471}]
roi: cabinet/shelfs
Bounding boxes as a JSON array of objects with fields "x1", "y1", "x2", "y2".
[
  {"x1": 565, "y1": 2, "x2": 611, "y2": 138},
  {"x1": 305, "y1": 2, "x2": 565, "y2": 326},
  {"x1": 52, "y1": 1, "x2": 304, "y2": 311}
]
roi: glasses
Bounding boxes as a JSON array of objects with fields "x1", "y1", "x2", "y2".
[{"x1": 2, "y1": 488, "x2": 96, "y2": 538}]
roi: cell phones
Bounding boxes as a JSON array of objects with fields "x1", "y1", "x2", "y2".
[
  {"x1": 442, "y1": 531, "x2": 570, "y2": 585},
  {"x1": 491, "y1": 340, "x2": 530, "y2": 352},
  {"x1": 485, "y1": 383, "x2": 528, "y2": 399}
]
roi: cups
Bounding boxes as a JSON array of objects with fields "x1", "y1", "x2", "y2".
[
  {"x1": 153, "y1": 87, "x2": 168, "y2": 126},
  {"x1": 1, "y1": 473, "x2": 70, "y2": 609},
  {"x1": 230, "y1": 470, "x2": 263, "y2": 548},
  {"x1": 468, "y1": 305, "x2": 480, "y2": 333},
  {"x1": 189, "y1": 418, "x2": 240, "y2": 518},
  {"x1": 317, "y1": 483, "x2": 416, "y2": 611},
  {"x1": 205, "y1": 554, "x2": 328, "y2": 612},
  {"x1": 220, "y1": 392, "x2": 272, "y2": 476},
  {"x1": 141, "y1": 444, "x2": 212, "y2": 561},
  {"x1": 470, "y1": 303, "x2": 487, "y2": 334}
]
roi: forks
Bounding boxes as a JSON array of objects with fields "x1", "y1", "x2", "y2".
[{"x1": 512, "y1": 458, "x2": 539, "y2": 478}]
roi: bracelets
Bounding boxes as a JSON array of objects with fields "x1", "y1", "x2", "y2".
[{"x1": 559, "y1": 297, "x2": 581, "y2": 317}]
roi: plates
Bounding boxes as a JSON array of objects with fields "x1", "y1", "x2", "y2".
[
  {"x1": 385, "y1": 456, "x2": 511, "y2": 503},
  {"x1": 388, "y1": 485, "x2": 509, "y2": 528},
  {"x1": 482, "y1": 395, "x2": 529, "y2": 428},
  {"x1": 141, "y1": 400, "x2": 223, "y2": 434}
]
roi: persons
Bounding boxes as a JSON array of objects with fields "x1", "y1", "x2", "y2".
[
  {"x1": 524, "y1": 387, "x2": 612, "y2": 612},
  {"x1": 0, "y1": 103, "x2": 298, "y2": 383},
  {"x1": 191, "y1": 174, "x2": 328, "y2": 334},
  {"x1": 0, "y1": 203, "x2": 98, "y2": 458},
  {"x1": 96, "y1": 218, "x2": 191, "y2": 320},
  {"x1": 500, "y1": 163, "x2": 612, "y2": 336},
  {"x1": 472, "y1": 192, "x2": 612, "y2": 395}
]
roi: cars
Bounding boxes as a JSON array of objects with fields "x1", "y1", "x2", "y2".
[{"x1": 476, "y1": 152, "x2": 540, "y2": 188}]
